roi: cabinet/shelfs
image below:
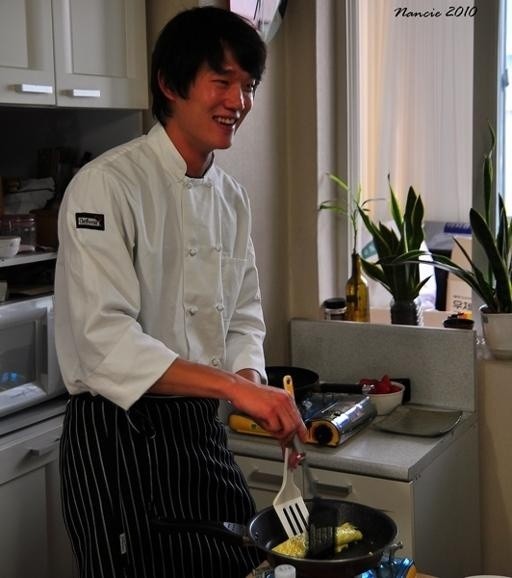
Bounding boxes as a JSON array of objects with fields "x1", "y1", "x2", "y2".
[
  {"x1": 2, "y1": 414, "x2": 82, "y2": 575},
  {"x1": 232, "y1": 421, "x2": 481, "y2": 578},
  {"x1": 0, "y1": 0, "x2": 150, "y2": 114}
]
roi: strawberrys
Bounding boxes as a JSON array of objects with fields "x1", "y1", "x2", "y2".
[{"x1": 359, "y1": 375, "x2": 400, "y2": 394}]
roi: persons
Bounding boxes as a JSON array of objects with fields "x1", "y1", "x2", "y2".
[{"x1": 54, "y1": 6, "x2": 310, "y2": 578}]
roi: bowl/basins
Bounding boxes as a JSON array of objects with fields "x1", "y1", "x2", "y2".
[
  {"x1": 0, "y1": 235, "x2": 22, "y2": 259},
  {"x1": 354, "y1": 380, "x2": 406, "y2": 416},
  {"x1": 372, "y1": 404, "x2": 463, "y2": 439}
]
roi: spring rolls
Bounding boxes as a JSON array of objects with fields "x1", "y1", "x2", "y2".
[{"x1": 272, "y1": 523, "x2": 362, "y2": 557}]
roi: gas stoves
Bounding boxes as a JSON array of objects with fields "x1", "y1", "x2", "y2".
[
  {"x1": 252, "y1": 540, "x2": 418, "y2": 578},
  {"x1": 228, "y1": 391, "x2": 378, "y2": 449}
]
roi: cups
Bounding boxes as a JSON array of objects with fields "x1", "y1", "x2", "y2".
[{"x1": 443, "y1": 319, "x2": 474, "y2": 330}]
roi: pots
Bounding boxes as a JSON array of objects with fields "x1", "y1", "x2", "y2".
[
  {"x1": 265, "y1": 365, "x2": 370, "y2": 403},
  {"x1": 149, "y1": 497, "x2": 399, "y2": 578}
]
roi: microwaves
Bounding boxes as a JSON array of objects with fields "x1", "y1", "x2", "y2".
[{"x1": 0, "y1": 291, "x2": 68, "y2": 420}]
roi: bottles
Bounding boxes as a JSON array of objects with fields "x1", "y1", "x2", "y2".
[
  {"x1": 344, "y1": 252, "x2": 370, "y2": 323},
  {"x1": 323, "y1": 297, "x2": 347, "y2": 320}
]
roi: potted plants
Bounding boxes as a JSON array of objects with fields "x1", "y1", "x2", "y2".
[
  {"x1": 431, "y1": 125, "x2": 512, "y2": 359},
  {"x1": 318, "y1": 168, "x2": 429, "y2": 326}
]
